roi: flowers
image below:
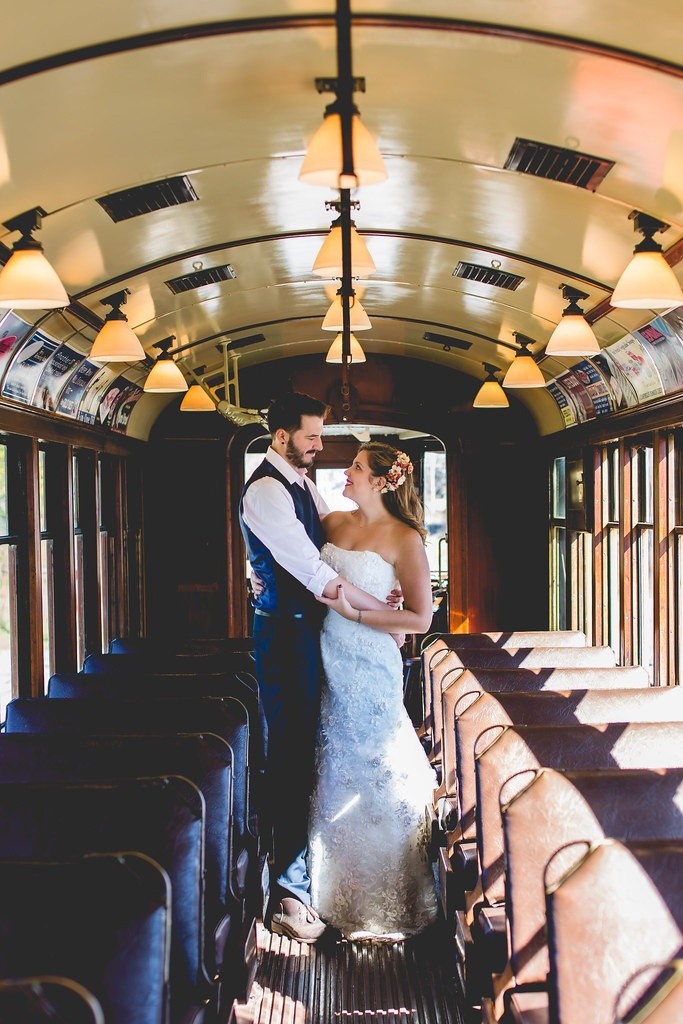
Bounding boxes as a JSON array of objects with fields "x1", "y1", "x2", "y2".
[{"x1": 381, "y1": 451, "x2": 416, "y2": 494}]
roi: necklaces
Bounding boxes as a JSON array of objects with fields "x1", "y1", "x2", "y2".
[{"x1": 359, "y1": 512, "x2": 390, "y2": 528}]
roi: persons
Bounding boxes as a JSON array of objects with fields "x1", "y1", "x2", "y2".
[{"x1": 238, "y1": 390, "x2": 438, "y2": 944}]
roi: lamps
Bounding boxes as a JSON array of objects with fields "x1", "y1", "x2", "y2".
[
  {"x1": 609, "y1": 210, "x2": 683, "y2": 309},
  {"x1": 297, "y1": 76, "x2": 387, "y2": 186},
  {"x1": 545, "y1": 283, "x2": 601, "y2": 356},
  {"x1": 326, "y1": 331, "x2": 366, "y2": 363},
  {"x1": 90, "y1": 288, "x2": 146, "y2": 362},
  {"x1": 472, "y1": 362, "x2": 509, "y2": 408},
  {"x1": 143, "y1": 335, "x2": 188, "y2": 393},
  {"x1": 0, "y1": 206, "x2": 70, "y2": 310},
  {"x1": 321, "y1": 278, "x2": 372, "y2": 330},
  {"x1": 502, "y1": 331, "x2": 546, "y2": 388},
  {"x1": 180, "y1": 365, "x2": 216, "y2": 411},
  {"x1": 311, "y1": 199, "x2": 376, "y2": 277}
]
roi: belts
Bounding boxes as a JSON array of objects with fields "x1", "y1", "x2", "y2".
[{"x1": 254, "y1": 608, "x2": 304, "y2": 618}]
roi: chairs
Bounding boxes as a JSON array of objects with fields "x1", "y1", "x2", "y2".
[
  {"x1": 414, "y1": 631, "x2": 683, "y2": 1023},
  {"x1": 0, "y1": 635, "x2": 277, "y2": 1024}
]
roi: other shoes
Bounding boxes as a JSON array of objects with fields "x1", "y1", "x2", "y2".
[{"x1": 271, "y1": 899, "x2": 336, "y2": 944}]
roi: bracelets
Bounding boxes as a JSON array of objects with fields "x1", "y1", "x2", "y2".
[{"x1": 356, "y1": 610, "x2": 362, "y2": 623}]
